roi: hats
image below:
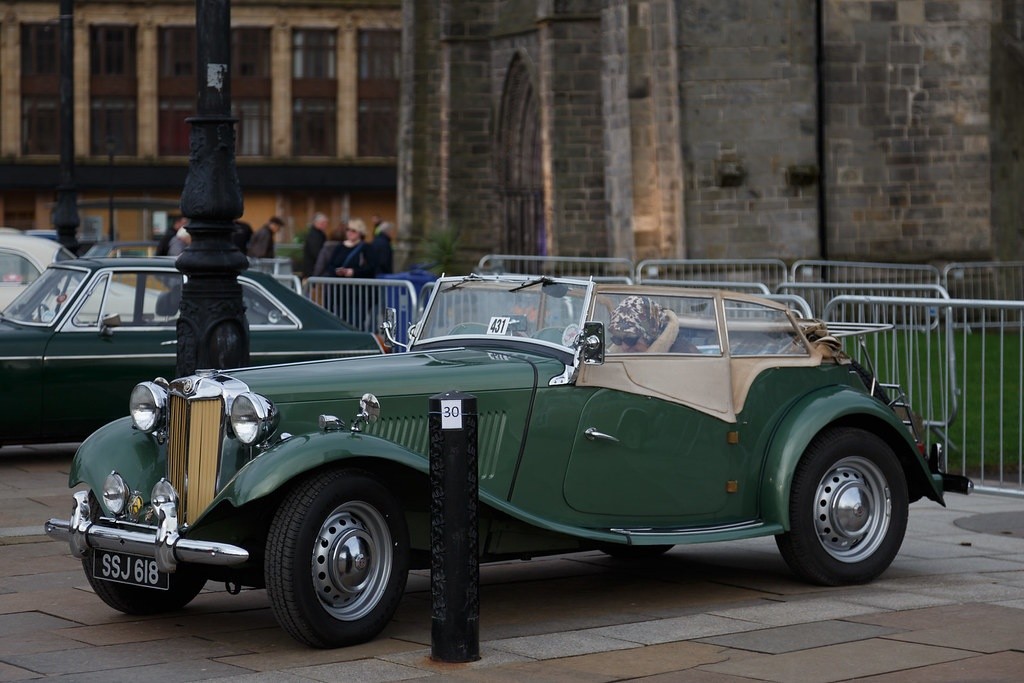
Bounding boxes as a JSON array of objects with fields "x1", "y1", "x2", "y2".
[
  {"x1": 270, "y1": 217, "x2": 284, "y2": 225},
  {"x1": 610, "y1": 297, "x2": 669, "y2": 344},
  {"x1": 348, "y1": 220, "x2": 367, "y2": 235}
]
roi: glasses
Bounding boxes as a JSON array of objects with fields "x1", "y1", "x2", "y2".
[
  {"x1": 346, "y1": 228, "x2": 356, "y2": 233},
  {"x1": 611, "y1": 336, "x2": 640, "y2": 346}
]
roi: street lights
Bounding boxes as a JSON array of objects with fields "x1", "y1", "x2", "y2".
[{"x1": 103, "y1": 133, "x2": 120, "y2": 242}]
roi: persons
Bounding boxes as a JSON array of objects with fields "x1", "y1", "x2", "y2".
[
  {"x1": 249, "y1": 214, "x2": 395, "y2": 277},
  {"x1": 607, "y1": 295, "x2": 701, "y2": 354},
  {"x1": 156, "y1": 215, "x2": 191, "y2": 257},
  {"x1": 324, "y1": 218, "x2": 377, "y2": 327}
]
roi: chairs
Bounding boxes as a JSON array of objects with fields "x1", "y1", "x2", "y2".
[
  {"x1": 155, "y1": 292, "x2": 179, "y2": 325},
  {"x1": 531, "y1": 326, "x2": 567, "y2": 348}
]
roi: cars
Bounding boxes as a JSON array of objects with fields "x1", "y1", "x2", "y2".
[
  {"x1": 0, "y1": 254, "x2": 394, "y2": 460},
  {"x1": 78, "y1": 240, "x2": 162, "y2": 258},
  {"x1": 44, "y1": 271, "x2": 973, "y2": 651},
  {"x1": 0, "y1": 234, "x2": 77, "y2": 318}
]
